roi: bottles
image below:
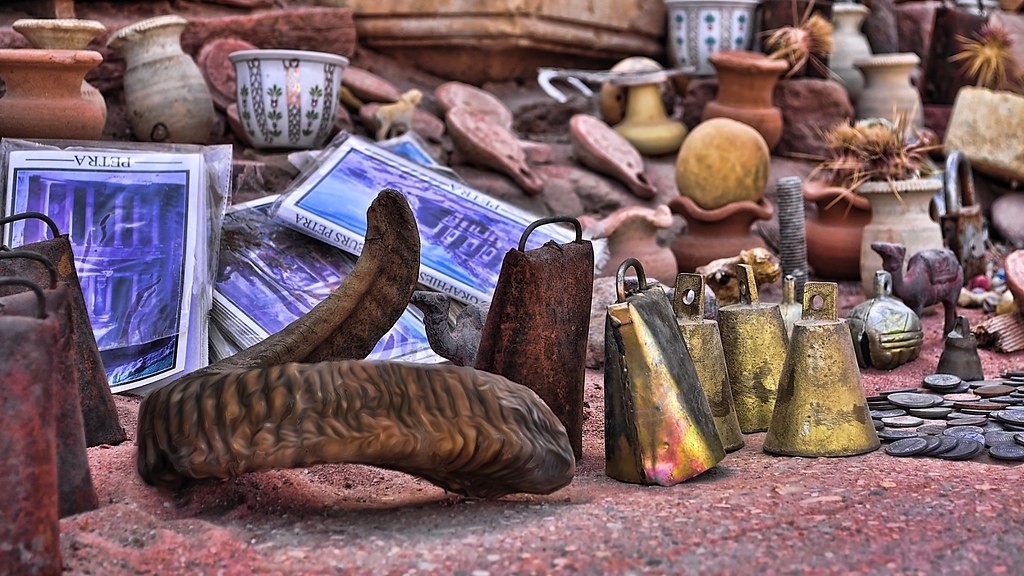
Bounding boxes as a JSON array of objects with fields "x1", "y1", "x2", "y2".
[
  {"x1": 856, "y1": 177, "x2": 944, "y2": 301},
  {"x1": 0, "y1": 49, "x2": 104, "y2": 141},
  {"x1": 106, "y1": 13, "x2": 214, "y2": 145},
  {"x1": 12, "y1": 18, "x2": 108, "y2": 130},
  {"x1": 701, "y1": 49, "x2": 790, "y2": 152},
  {"x1": 827, "y1": 3, "x2": 874, "y2": 99},
  {"x1": 852, "y1": 51, "x2": 923, "y2": 146}
]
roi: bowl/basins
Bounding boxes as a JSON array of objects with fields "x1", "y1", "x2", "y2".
[
  {"x1": 228, "y1": 48, "x2": 349, "y2": 149},
  {"x1": 664, "y1": 1, "x2": 760, "y2": 76},
  {"x1": 226, "y1": 102, "x2": 252, "y2": 147}
]
